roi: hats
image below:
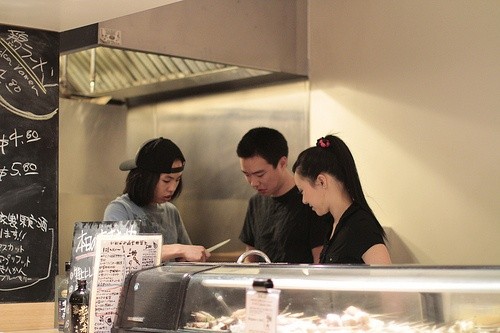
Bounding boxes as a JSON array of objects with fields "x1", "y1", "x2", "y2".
[{"x1": 119, "y1": 137, "x2": 185, "y2": 173}]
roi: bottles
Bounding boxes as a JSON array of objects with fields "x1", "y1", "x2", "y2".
[
  {"x1": 58, "y1": 261, "x2": 71, "y2": 331},
  {"x1": 69, "y1": 280, "x2": 93, "y2": 333}
]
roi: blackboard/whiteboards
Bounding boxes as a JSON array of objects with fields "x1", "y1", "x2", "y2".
[
  {"x1": 0, "y1": 23, "x2": 59, "y2": 305},
  {"x1": 64, "y1": 219, "x2": 142, "y2": 333}
]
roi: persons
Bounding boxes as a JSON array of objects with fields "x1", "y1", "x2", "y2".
[
  {"x1": 102, "y1": 136, "x2": 211, "y2": 267},
  {"x1": 235, "y1": 127, "x2": 334, "y2": 264},
  {"x1": 292, "y1": 134, "x2": 392, "y2": 265}
]
